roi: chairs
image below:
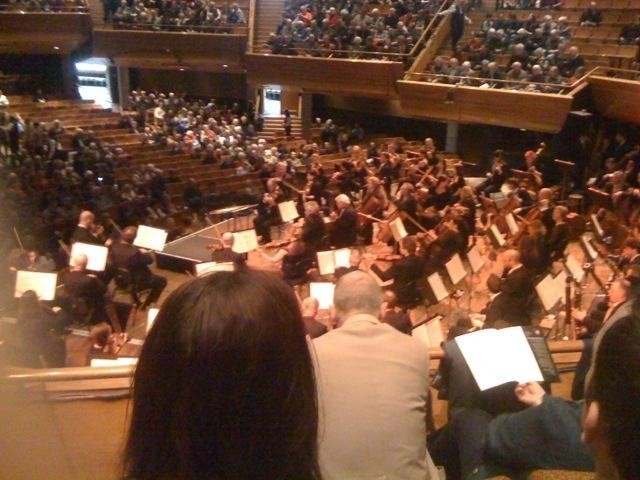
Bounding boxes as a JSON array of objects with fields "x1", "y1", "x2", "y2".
[{"x1": 0, "y1": 0, "x2": 640, "y2": 366}]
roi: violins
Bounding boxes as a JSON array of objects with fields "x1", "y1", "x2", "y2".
[
  {"x1": 329, "y1": 208, "x2": 346, "y2": 218},
  {"x1": 264, "y1": 188, "x2": 283, "y2": 204},
  {"x1": 358, "y1": 254, "x2": 404, "y2": 263},
  {"x1": 253, "y1": 239, "x2": 290, "y2": 251},
  {"x1": 206, "y1": 243, "x2": 223, "y2": 251}
]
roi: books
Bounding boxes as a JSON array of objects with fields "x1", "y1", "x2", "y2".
[{"x1": 453, "y1": 324, "x2": 546, "y2": 393}]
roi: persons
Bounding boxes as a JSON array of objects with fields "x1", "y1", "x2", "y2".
[
  {"x1": 308, "y1": 271, "x2": 442, "y2": 480},
  {"x1": 121, "y1": 267, "x2": 325, "y2": 480},
  {"x1": 262, "y1": 1, "x2": 451, "y2": 70},
  {"x1": 101, "y1": 1, "x2": 247, "y2": 34},
  {"x1": 1, "y1": 89, "x2": 168, "y2": 250},
  {"x1": 123, "y1": 89, "x2": 265, "y2": 168},
  {"x1": 576, "y1": 315, "x2": 639, "y2": 480},
  {"x1": 263, "y1": 109, "x2": 640, "y2": 353},
  {"x1": 1, "y1": 210, "x2": 166, "y2": 354},
  {"x1": 617, "y1": 22, "x2": 639, "y2": 45},
  {"x1": 419, "y1": 1, "x2": 603, "y2": 94},
  {"x1": 426, "y1": 374, "x2": 594, "y2": 480},
  {"x1": 1, "y1": 1, "x2": 87, "y2": 13}
]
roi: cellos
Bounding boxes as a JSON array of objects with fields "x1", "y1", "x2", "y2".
[
  {"x1": 355, "y1": 180, "x2": 384, "y2": 232},
  {"x1": 508, "y1": 143, "x2": 546, "y2": 196},
  {"x1": 377, "y1": 186, "x2": 429, "y2": 241},
  {"x1": 599, "y1": 168, "x2": 632, "y2": 246},
  {"x1": 514, "y1": 184, "x2": 562, "y2": 245}
]
